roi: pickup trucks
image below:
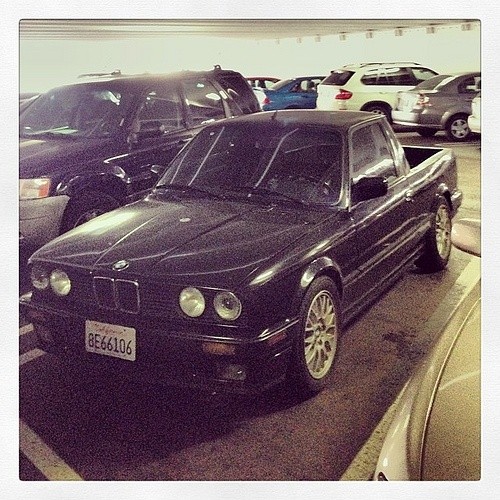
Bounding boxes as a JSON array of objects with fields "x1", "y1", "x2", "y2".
[{"x1": 22, "y1": 106, "x2": 464, "y2": 399}]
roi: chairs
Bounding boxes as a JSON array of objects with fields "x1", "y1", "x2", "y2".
[
  {"x1": 457, "y1": 77, "x2": 475, "y2": 92},
  {"x1": 286, "y1": 144, "x2": 344, "y2": 203},
  {"x1": 76, "y1": 99, "x2": 120, "y2": 137},
  {"x1": 134, "y1": 100, "x2": 183, "y2": 134},
  {"x1": 307, "y1": 81, "x2": 317, "y2": 93},
  {"x1": 215, "y1": 148, "x2": 287, "y2": 193}
]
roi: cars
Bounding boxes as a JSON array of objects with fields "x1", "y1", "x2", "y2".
[
  {"x1": 394, "y1": 71, "x2": 480, "y2": 140},
  {"x1": 256, "y1": 73, "x2": 327, "y2": 112}
]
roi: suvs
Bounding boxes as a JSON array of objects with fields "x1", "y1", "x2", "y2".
[
  {"x1": 315, "y1": 61, "x2": 440, "y2": 130},
  {"x1": 21, "y1": 72, "x2": 250, "y2": 279}
]
